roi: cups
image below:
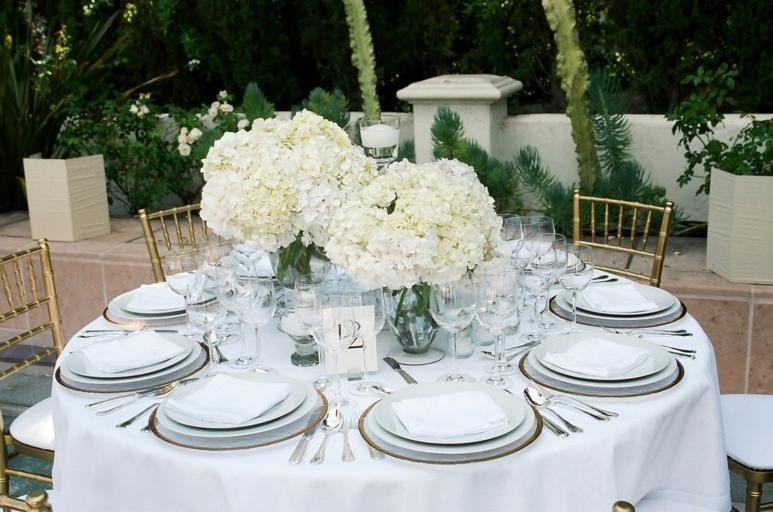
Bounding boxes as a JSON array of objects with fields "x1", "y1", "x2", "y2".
[{"x1": 358, "y1": 114, "x2": 401, "y2": 164}]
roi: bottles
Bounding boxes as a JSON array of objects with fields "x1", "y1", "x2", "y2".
[{"x1": 549, "y1": 294, "x2": 688, "y2": 329}]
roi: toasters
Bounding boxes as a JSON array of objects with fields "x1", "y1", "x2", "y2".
[{"x1": 166, "y1": 248, "x2": 205, "y2": 339}]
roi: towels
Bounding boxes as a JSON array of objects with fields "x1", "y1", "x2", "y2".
[
  {"x1": 391, "y1": 390, "x2": 508, "y2": 439},
  {"x1": 165, "y1": 373, "x2": 292, "y2": 426},
  {"x1": 544, "y1": 332, "x2": 650, "y2": 376},
  {"x1": 126, "y1": 284, "x2": 186, "y2": 311},
  {"x1": 498, "y1": 239, "x2": 552, "y2": 260},
  {"x1": 578, "y1": 284, "x2": 658, "y2": 313},
  {"x1": 80, "y1": 330, "x2": 186, "y2": 375}
]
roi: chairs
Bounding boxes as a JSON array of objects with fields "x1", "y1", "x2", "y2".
[
  {"x1": 138, "y1": 203, "x2": 233, "y2": 283},
  {"x1": 720, "y1": 393, "x2": 773, "y2": 512},
  {"x1": 572, "y1": 188, "x2": 675, "y2": 288},
  {"x1": 0, "y1": 238, "x2": 65, "y2": 512}
]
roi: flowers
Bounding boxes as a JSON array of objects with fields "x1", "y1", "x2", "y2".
[
  {"x1": 199, "y1": 108, "x2": 379, "y2": 283},
  {"x1": 323, "y1": 157, "x2": 504, "y2": 329}
]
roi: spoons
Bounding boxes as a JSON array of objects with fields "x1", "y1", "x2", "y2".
[
  {"x1": 310, "y1": 408, "x2": 343, "y2": 466},
  {"x1": 483, "y1": 338, "x2": 540, "y2": 360},
  {"x1": 94, "y1": 381, "x2": 178, "y2": 420},
  {"x1": 204, "y1": 330, "x2": 226, "y2": 363},
  {"x1": 524, "y1": 386, "x2": 585, "y2": 435}
]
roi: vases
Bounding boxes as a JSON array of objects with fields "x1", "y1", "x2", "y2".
[
  {"x1": 274, "y1": 234, "x2": 331, "y2": 309},
  {"x1": 388, "y1": 281, "x2": 445, "y2": 366}
]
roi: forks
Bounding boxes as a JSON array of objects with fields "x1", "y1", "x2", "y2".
[
  {"x1": 348, "y1": 405, "x2": 388, "y2": 462},
  {"x1": 599, "y1": 322, "x2": 688, "y2": 334},
  {"x1": 527, "y1": 379, "x2": 620, "y2": 418}
]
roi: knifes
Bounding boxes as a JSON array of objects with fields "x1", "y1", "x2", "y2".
[
  {"x1": 85, "y1": 377, "x2": 201, "y2": 410},
  {"x1": 289, "y1": 405, "x2": 326, "y2": 466},
  {"x1": 384, "y1": 357, "x2": 418, "y2": 385}
]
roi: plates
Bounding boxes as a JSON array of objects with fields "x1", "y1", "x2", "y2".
[
  {"x1": 554, "y1": 293, "x2": 681, "y2": 322},
  {"x1": 60, "y1": 339, "x2": 202, "y2": 385},
  {"x1": 55, "y1": 341, "x2": 211, "y2": 393},
  {"x1": 358, "y1": 398, "x2": 544, "y2": 464},
  {"x1": 533, "y1": 333, "x2": 670, "y2": 381},
  {"x1": 187, "y1": 289, "x2": 234, "y2": 310},
  {"x1": 564, "y1": 283, "x2": 674, "y2": 316},
  {"x1": 518, "y1": 349, "x2": 687, "y2": 399},
  {"x1": 148, "y1": 387, "x2": 330, "y2": 451},
  {"x1": 493, "y1": 240, "x2": 586, "y2": 274},
  {"x1": 65, "y1": 331, "x2": 193, "y2": 377},
  {"x1": 102, "y1": 307, "x2": 186, "y2": 328},
  {"x1": 367, "y1": 403, "x2": 536, "y2": 455},
  {"x1": 118, "y1": 286, "x2": 186, "y2": 315},
  {"x1": 107, "y1": 291, "x2": 188, "y2": 321},
  {"x1": 371, "y1": 380, "x2": 526, "y2": 445},
  {"x1": 155, "y1": 386, "x2": 317, "y2": 439},
  {"x1": 237, "y1": 274, "x2": 277, "y2": 282},
  {"x1": 162, "y1": 371, "x2": 306, "y2": 430},
  {"x1": 528, "y1": 348, "x2": 677, "y2": 390}
]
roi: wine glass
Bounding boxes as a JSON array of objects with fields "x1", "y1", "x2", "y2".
[
  {"x1": 501, "y1": 310, "x2": 520, "y2": 378},
  {"x1": 234, "y1": 267, "x2": 278, "y2": 376},
  {"x1": 429, "y1": 270, "x2": 477, "y2": 387},
  {"x1": 199, "y1": 235, "x2": 238, "y2": 332},
  {"x1": 514, "y1": 243, "x2": 557, "y2": 343},
  {"x1": 185, "y1": 278, "x2": 229, "y2": 380},
  {"x1": 474, "y1": 275, "x2": 524, "y2": 389},
  {"x1": 520, "y1": 215, "x2": 556, "y2": 265},
  {"x1": 485, "y1": 255, "x2": 530, "y2": 376},
  {"x1": 310, "y1": 281, "x2": 357, "y2": 410},
  {"x1": 273, "y1": 292, "x2": 336, "y2": 366},
  {"x1": 490, "y1": 213, "x2": 524, "y2": 261},
  {"x1": 532, "y1": 232, "x2": 567, "y2": 332},
  {"x1": 216, "y1": 262, "x2": 262, "y2": 369},
  {"x1": 293, "y1": 272, "x2": 341, "y2": 394},
  {"x1": 556, "y1": 244, "x2": 592, "y2": 334},
  {"x1": 340, "y1": 277, "x2": 387, "y2": 398}
]
